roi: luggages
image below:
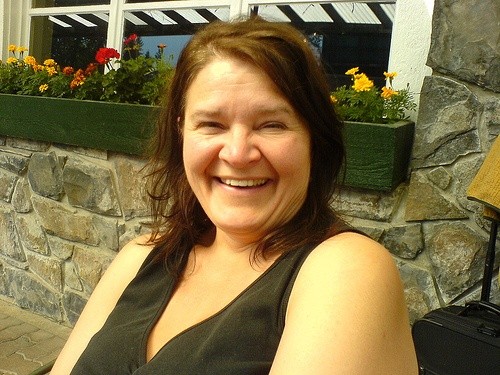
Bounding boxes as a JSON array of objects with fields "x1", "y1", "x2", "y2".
[{"x1": 412, "y1": 219, "x2": 500, "y2": 375}]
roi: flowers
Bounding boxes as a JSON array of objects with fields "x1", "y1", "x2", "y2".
[{"x1": 0, "y1": 34, "x2": 417, "y2": 124}]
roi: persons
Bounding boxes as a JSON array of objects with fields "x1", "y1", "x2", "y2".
[{"x1": 48, "y1": 14, "x2": 419, "y2": 375}]
